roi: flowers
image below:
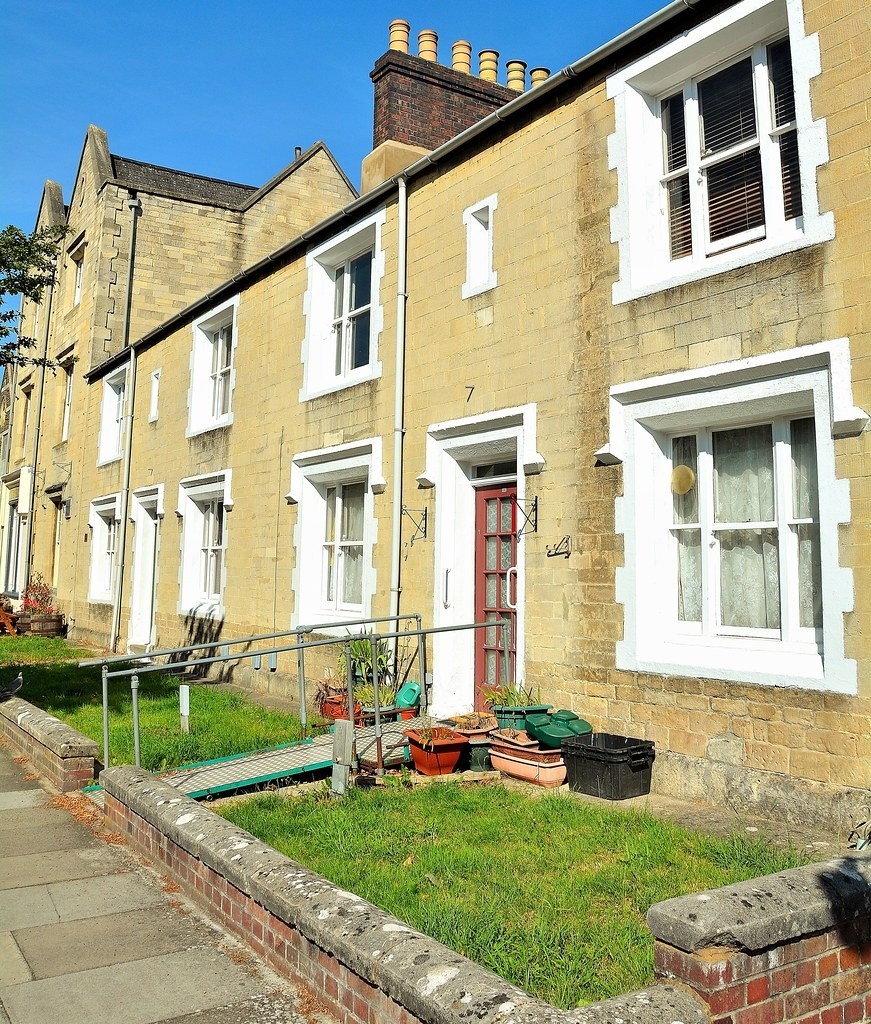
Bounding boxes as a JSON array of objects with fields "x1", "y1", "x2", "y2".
[{"x1": 17, "y1": 571, "x2": 59, "y2": 614}]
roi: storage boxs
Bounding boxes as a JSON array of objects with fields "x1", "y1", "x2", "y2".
[{"x1": 562, "y1": 732, "x2": 656, "y2": 800}]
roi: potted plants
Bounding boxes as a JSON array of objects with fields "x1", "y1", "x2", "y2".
[
  {"x1": 312, "y1": 679, "x2": 349, "y2": 719},
  {"x1": 330, "y1": 643, "x2": 361, "y2": 726},
  {"x1": 330, "y1": 625, "x2": 395, "y2": 685},
  {"x1": 353, "y1": 680, "x2": 397, "y2": 727},
  {"x1": 403, "y1": 706, "x2": 469, "y2": 776},
  {"x1": 475, "y1": 676, "x2": 554, "y2": 730}
]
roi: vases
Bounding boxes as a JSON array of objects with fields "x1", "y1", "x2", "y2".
[
  {"x1": 489, "y1": 728, "x2": 540, "y2": 750},
  {"x1": 468, "y1": 738, "x2": 493, "y2": 772},
  {"x1": 30, "y1": 614, "x2": 63, "y2": 637},
  {"x1": 16, "y1": 614, "x2": 30, "y2": 635},
  {"x1": 490, "y1": 739, "x2": 562, "y2": 763},
  {"x1": 396, "y1": 680, "x2": 422, "y2": 722},
  {"x1": 488, "y1": 747, "x2": 567, "y2": 788}
]
